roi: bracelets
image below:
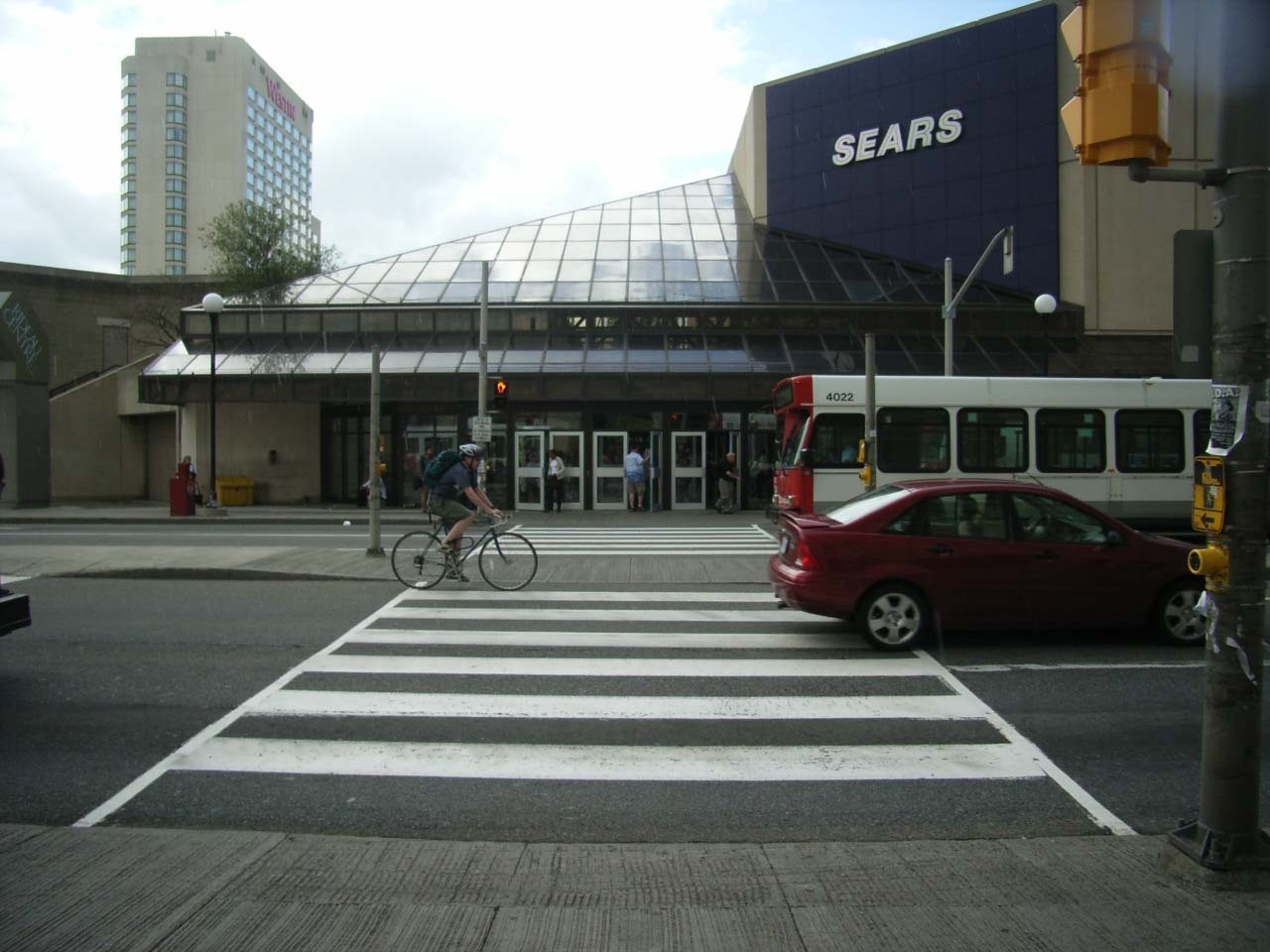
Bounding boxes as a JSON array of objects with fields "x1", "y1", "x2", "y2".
[{"x1": 491, "y1": 506, "x2": 495, "y2": 509}]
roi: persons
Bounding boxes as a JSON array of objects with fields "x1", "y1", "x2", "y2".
[
  {"x1": 958, "y1": 429, "x2": 1002, "y2": 470},
  {"x1": 714, "y1": 451, "x2": 742, "y2": 515},
  {"x1": 545, "y1": 449, "x2": 566, "y2": 516},
  {"x1": 175, "y1": 456, "x2": 200, "y2": 496},
  {"x1": 623, "y1": 444, "x2": 650, "y2": 513},
  {"x1": 362, "y1": 475, "x2": 387, "y2": 508},
  {"x1": 427, "y1": 444, "x2": 503, "y2": 582},
  {"x1": 678, "y1": 444, "x2": 702, "y2": 503},
  {"x1": 816, "y1": 434, "x2": 857, "y2": 468},
  {"x1": 758, "y1": 464, "x2": 778, "y2": 524},
  {"x1": 421, "y1": 444, "x2": 437, "y2": 513},
  {"x1": 956, "y1": 497, "x2": 978, "y2": 539}
]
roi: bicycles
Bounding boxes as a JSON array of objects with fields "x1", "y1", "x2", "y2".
[{"x1": 392, "y1": 503, "x2": 538, "y2": 592}]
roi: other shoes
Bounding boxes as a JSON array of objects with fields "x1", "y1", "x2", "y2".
[
  {"x1": 446, "y1": 569, "x2": 470, "y2": 582},
  {"x1": 629, "y1": 508, "x2": 636, "y2": 512},
  {"x1": 436, "y1": 544, "x2": 454, "y2": 553},
  {"x1": 638, "y1": 507, "x2": 645, "y2": 511}
]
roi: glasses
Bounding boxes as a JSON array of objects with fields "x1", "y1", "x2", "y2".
[{"x1": 476, "y1": 458, "x2": 481, "y2": 461}]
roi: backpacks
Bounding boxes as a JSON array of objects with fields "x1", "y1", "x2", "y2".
[{"x1": 421, "y1": 449, "x2": 464, "y2": 488}]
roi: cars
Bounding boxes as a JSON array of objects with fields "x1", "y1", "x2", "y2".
[{"x1": 771, "y1": 475, "x2": 1220, "y2": 652}]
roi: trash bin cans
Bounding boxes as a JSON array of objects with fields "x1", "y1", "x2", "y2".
[{"x1": 170, "y1": 462, "x2": 195, "y2": 516}]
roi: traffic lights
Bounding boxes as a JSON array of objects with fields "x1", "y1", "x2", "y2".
[
  {"x1": 494, "y1": 380, "x2": 508, "y2": 396},
  {"x1": 378, "y1": 458, "x2": 389, "y2": 476}
]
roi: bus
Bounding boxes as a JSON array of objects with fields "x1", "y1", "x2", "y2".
[{"x1": 765, "y1": 373, "x2": 1214, "y2": 536}]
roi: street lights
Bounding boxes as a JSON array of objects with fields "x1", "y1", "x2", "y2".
[
  {"x1": 202, "y1": 292, "x2": 224, "y2": 510},
  {"x1": 1034, "y1": 294, "x2": 1056, "y2": 377}
]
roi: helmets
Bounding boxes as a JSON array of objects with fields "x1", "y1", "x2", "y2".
[{"x1": 459, "y1": 444, "x2": 483, "y2": 457}]
